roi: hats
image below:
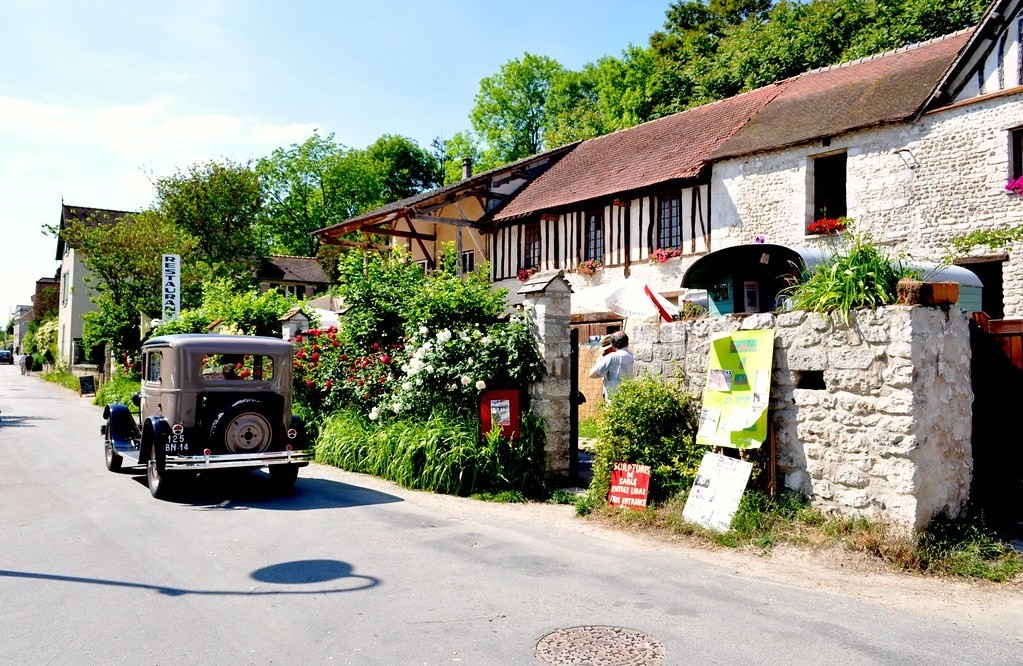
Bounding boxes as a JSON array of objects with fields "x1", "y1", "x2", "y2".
[{"x1": 598, "y1": 334, "x2": 613, "y2": 352}]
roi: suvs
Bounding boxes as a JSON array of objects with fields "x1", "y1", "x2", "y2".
[{"x1": 102, "y1": 333, "x2": 316, "y2": 499}]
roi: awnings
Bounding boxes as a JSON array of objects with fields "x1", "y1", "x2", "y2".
[{"x1": 311, "y1": 136, "x2": 585, "y2": 277}]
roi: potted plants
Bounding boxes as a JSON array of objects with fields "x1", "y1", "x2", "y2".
[{"x1": 896, "y1": 224, "x2": 1023, "y2": 305}]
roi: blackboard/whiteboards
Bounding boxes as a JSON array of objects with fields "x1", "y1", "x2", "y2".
[{"x1": 79, "y1": 375, "x2": 96, "y2": 395}]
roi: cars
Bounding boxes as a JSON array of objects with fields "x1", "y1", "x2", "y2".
[{"x1": 0, "y1": 350, "x2": 14, "y2": 365}]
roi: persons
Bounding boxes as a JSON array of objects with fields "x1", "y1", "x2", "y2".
[
  {"x1": 15, "y1": 346, "x2": 19, "y2": 355},
  {"x1": 19, "y1": 353, "x2": 34, "y2": 376},
  {"x1": 588, "y1": 331, "x2": 635, "y2": 413},
  {"x1": 10, "y1": 346, "x2": 14, "y2": 355}
]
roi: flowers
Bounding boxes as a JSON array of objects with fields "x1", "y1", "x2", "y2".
[
  {"x1": 648, "y1": 247, "x2": 681, "y2": 267},
  {"x1": 577, "y1": 259, "x2": 602, "y2": 280},
  {"x1": 807, "y1": 215, "x2": 845, "y2": 235},
  {"x1": 1005, "y1": 176, "x2": 1023, "y2": 197},
  {"x1": 516, "y1": 266, "x2": 537, "y2": 282}
]
